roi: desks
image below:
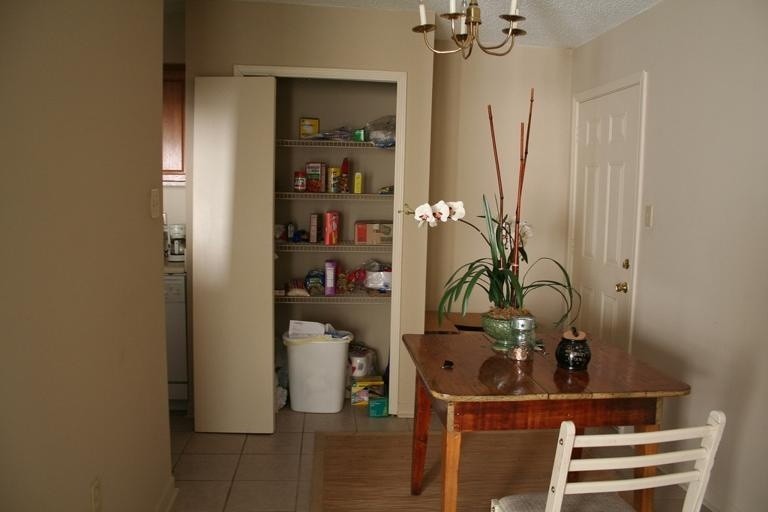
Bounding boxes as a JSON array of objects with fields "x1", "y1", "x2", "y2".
[
  {"x1": 445, "y1": 311, "x2": 486, "y2": 328},
  {"x1": 401, "y1": 331, "x2": 693, "y2": 511},
  {"x1": 424, "y1": 310, "x2": 458, "y2": 333}
]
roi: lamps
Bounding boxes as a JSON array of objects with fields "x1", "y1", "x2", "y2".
[{"x1": 410, "y1": 2, "x2": 530, "y2": 64}]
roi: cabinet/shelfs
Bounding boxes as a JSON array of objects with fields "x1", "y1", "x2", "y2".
[
  {"x1": 274, "y1": 136, "x2": 396, "y2": 304},
  {"x1": 161, "y1": 62, "x2": 185, "y2": 177},
  {"x1": 163, "y1": 272, "x2": 189, "y2": 414}
]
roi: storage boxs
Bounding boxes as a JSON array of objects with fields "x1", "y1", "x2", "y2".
[
  {"x1": 362, "y1": 259, "x2": 393, "y2": 291},
  {"x1": 350, "y1": 375, "x2": 386, "y2": 407},
  {"x1": 354, "y1": 219, "x2": 393, "y2": 244}
]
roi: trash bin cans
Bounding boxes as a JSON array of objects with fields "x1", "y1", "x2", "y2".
[{"x1": 283, "y1": 330, "x2": 354, "y2": 414}]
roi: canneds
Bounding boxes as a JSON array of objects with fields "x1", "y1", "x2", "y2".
[
  {"x1": 323, "y1": 210, "x2": 341, "y2": 245},
  {"x1": 326, "y1": 167, "x2": 341, "y2": 193}
]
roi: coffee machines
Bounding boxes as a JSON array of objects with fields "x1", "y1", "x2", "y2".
[{"x1": 167, "y1": 224, "x2": 186, "y2": 262}]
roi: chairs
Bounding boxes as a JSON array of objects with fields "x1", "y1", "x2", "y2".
[{"x1": 486, "y1": 409, "x2": 729, "y2": 511}]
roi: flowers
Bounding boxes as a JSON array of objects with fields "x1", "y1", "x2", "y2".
[{"x1": 396, "y1": 87, "x2": 583, "y2": 328}]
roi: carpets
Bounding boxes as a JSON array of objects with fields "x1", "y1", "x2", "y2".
[{"x1": 309, "y1": 425, "x2": 655, "y2": 512}]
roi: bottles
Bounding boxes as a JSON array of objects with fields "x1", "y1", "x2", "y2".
[
  {"x1": 556, "y1": 328, "x2": 590, "y2": 371},
  {"x1": 338, "y1": 158, "x2": 351, "y2": 193}
]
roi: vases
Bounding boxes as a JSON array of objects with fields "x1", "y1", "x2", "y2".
[{"x1": 476, "y1": 306, "x2": 538, "y2": 357}]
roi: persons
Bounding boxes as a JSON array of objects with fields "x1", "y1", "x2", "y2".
[
  {"x1": 329, "y1": 214, "x2": 339, "y2": 245},
  {"x1": 329, "y1": 171, "x2": 340, "y2": 189}
]
